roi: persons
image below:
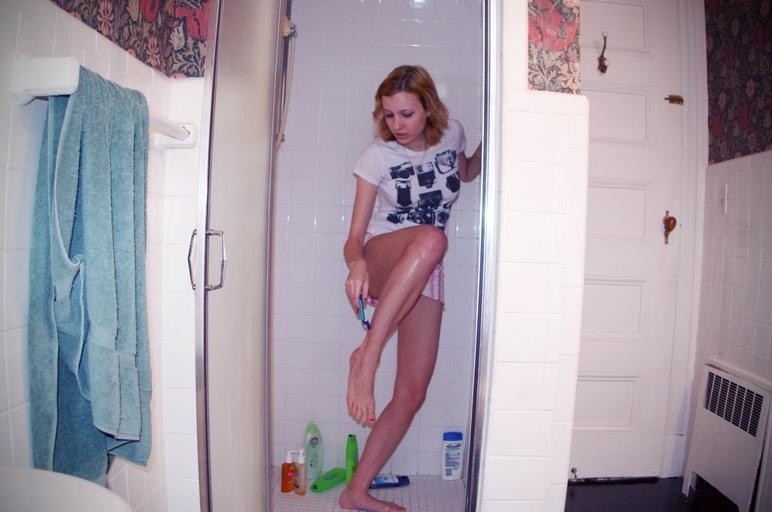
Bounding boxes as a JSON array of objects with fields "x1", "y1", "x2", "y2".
[{"x1": 339, "y1": 64, "x2": 484, "y2": 512}]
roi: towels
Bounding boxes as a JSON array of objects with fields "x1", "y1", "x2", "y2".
[{"x1": 26, "y1": 65, "x2": 152, "y2": 487}]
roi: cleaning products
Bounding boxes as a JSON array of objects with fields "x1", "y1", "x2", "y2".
[
  {"x1": 282, "y1": 451, "x2": 297, "y2": 493},
  {"x1": 294, "y1": 450, "x2": 305, "y2": 495}
]
